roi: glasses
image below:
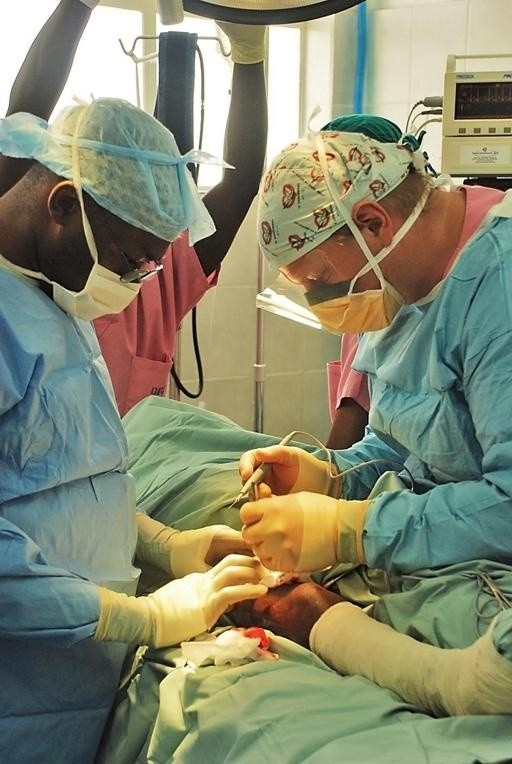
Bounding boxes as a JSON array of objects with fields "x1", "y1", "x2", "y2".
[{"x1": 100, "y1": 208, "x2": 163, "y2": 284}]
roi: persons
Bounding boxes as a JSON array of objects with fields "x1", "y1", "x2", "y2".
[
  {"x1": 2, "y1": 94, "x2": 274, "y2": 763},
  {"x1": 195, "y1": 555, "x2": 511, "y2": 723},
  {"x1": 318, "y1": 112, "x2": 411, "y2": 449},
  {"x1": 1, "y1": 0, "x2": 273, "y2": 426},
  {"x1": 236, "y1": 111, "x2": 512, "y2": 581}
]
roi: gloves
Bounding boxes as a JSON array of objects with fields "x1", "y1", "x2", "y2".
[
  {"x1": 130, "y1": 508, "x2": 268, "y2": 602},
  {"x1": 214, "y1": 18, "x2": 265, "y2": 65},
  {"x1": 94, "y1": 552, "x2": 268, "y2": 651},
  {"x1": 237, "y1": 444, "x2": 369, "y2": 574}
]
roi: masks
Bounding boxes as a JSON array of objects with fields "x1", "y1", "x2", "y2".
[
  {"x1": 53, "y1": 263, "x2": 143, "y2": 320},
  {"x1": 304, "y1": 278, "x2": 405, "y2": 335}
]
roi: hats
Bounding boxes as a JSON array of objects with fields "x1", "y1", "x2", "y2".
[{"x1": 255, "y1": 112, "x2": 428, "y2": 269}]
